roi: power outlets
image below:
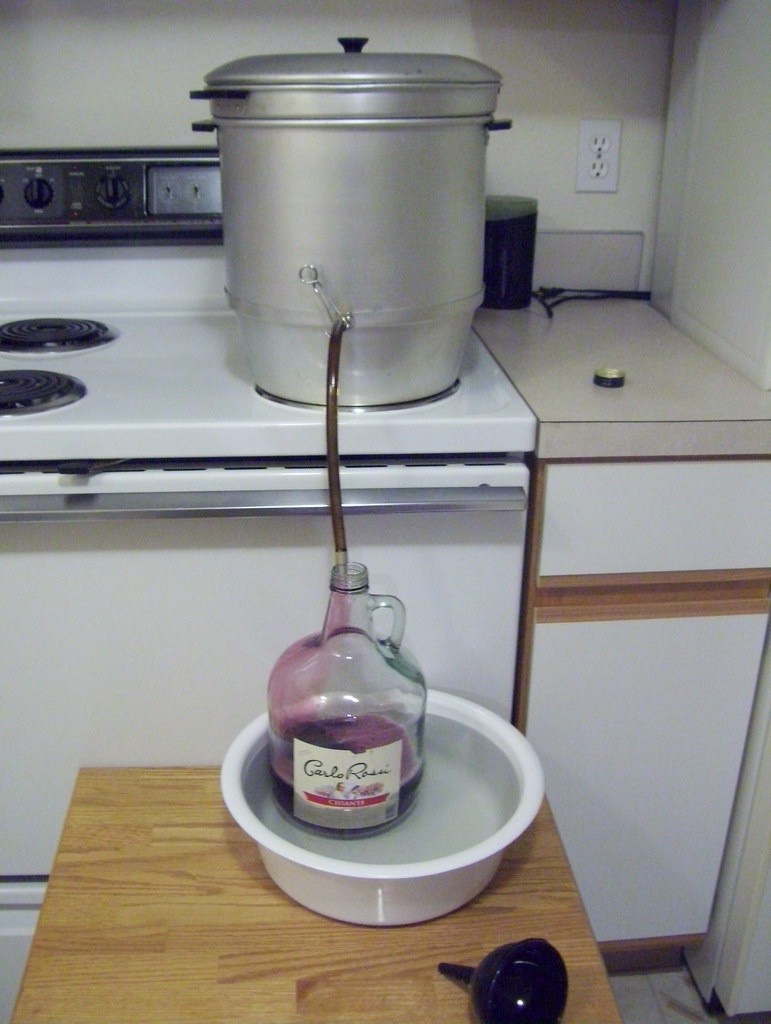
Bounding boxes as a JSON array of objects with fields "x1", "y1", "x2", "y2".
[{"x1": 574, "y1": 118, "x2": 619, "y2": 194}]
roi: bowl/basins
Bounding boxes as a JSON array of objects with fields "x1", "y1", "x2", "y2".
[{"x1": 219, "y1": 689, "x2": 545, "y2": 929}]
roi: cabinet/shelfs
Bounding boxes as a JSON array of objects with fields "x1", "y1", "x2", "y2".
[{"x1": 530, "y1": 460, "x2": 769, "y2": 976}]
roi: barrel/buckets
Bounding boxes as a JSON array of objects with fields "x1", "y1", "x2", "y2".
[{"x1": 482, "y1": 194, "x2": 537, "y2": 309}]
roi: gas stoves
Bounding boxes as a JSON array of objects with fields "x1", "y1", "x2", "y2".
[{"x1": 0, "y1": 146, "x2": 542, "y2": 477}]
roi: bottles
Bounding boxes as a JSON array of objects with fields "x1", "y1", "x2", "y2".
[{"x1": 268, "y1": 562, "x2": 425, "y2": 841}]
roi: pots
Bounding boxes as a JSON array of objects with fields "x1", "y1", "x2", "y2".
[{"x1": 190, "y1": 32, "x2": 517, "y2": 408}]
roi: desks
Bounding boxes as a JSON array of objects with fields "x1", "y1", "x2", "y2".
[{"x1": 12, "y1": 765, "x2": 623, "y2": 1024}]
